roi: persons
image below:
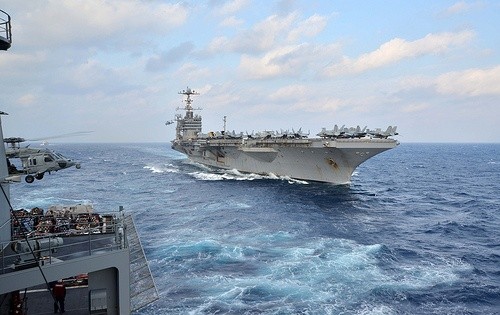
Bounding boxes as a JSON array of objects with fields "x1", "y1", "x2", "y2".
[{"x1": 47, "y1": 278, "x2": 71, "y2": 315}]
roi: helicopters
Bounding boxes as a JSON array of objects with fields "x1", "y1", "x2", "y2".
[{"x1": 2, "y1": 131, "x2": 90, "y2": 184}]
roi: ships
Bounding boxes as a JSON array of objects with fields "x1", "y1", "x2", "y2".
[
  {"x1": 165, "y1": 86, "x2": 401, "y2": 185},
  {"x1": 0, "y1": 8, "x2": 159, "y2": 315}
]
